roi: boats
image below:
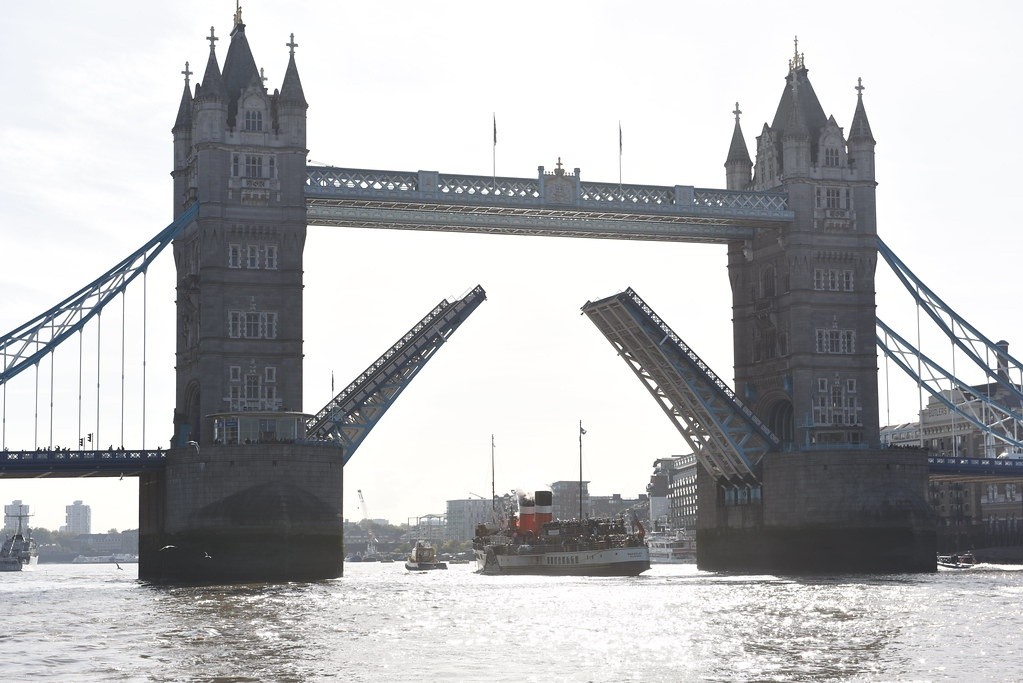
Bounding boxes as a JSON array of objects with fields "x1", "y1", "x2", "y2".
[
  {"x1": 471, "y1": 489, "x2": 652, "y2": 575},
  {"x1": 0, "y1": 552, "x2": 23, "y2": 571},
  {"x1": 646, "y1": 528, "x2": 698, "y2": 565},
  {"x1": 935, "y1": 550, "x2": 976, "y2": 569},
  {"x1": 0, "y1": 505, "x2": 40, "y2": 567},
  {"x1": 405, "y1": 539, "x2": 448, "y2": 570}
]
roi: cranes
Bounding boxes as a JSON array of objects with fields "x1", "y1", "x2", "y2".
[{"x1": 356, "y1": 489, "x2": 369, "y2": 520}]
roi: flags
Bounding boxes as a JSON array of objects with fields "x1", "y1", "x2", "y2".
[{"x1": 581, "y1": 427, "x2": 587, "y2": 434}]
